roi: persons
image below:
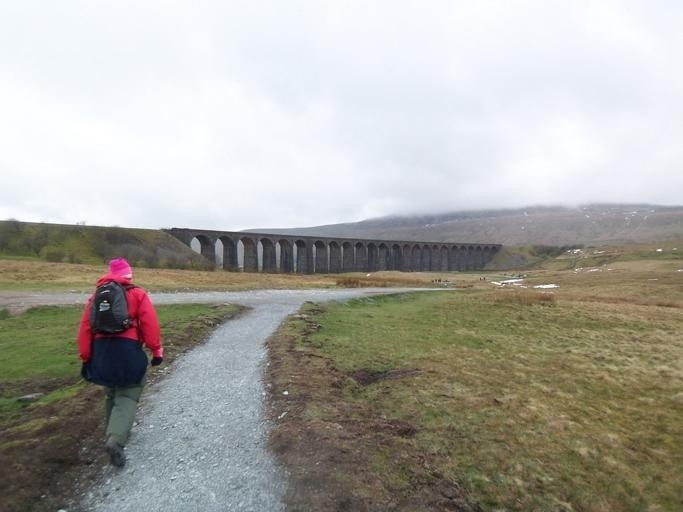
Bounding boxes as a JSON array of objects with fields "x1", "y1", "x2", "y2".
[{"x1": 76, "y1": 257, "x2": 163, "y2": 467}]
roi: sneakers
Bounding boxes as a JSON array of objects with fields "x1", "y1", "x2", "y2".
[{"x1": 104, "y1": 441, "x2": 126, "y2": 466}]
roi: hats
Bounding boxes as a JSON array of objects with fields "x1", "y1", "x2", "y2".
[{"x1": 109, "y1": 258, "x2": 132, "y2": 278}]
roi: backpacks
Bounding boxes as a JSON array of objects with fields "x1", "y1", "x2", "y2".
[{"x1": 89, "y1": 281, "x2": 138, "y2": 334}]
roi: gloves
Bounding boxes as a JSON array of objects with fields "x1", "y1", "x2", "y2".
[{"x1": 150, "y1": 357, "x2": 163, "y2": 367}]
